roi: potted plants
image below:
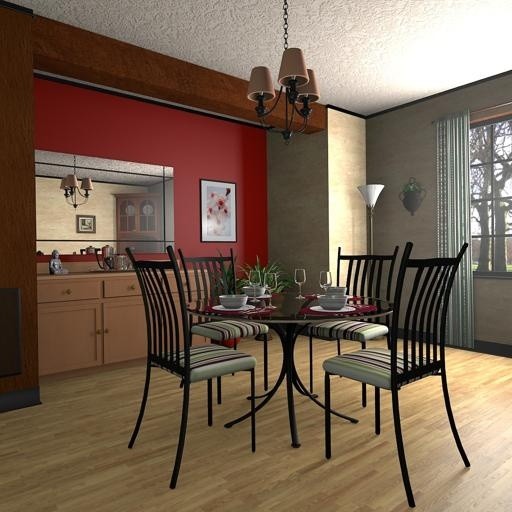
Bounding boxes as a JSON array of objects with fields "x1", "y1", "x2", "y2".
[
  {"x1": 237, "y1": 255, "x2": 294, "y2": 329},
  {"x1": 397, "y1": 176, "x2": 427, "y2": 217},
  {"x1": 208, "y1": 249, "x2": 241, "y2": 348}
]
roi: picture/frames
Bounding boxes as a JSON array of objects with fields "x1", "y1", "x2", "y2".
[
  {"x1": 75, "y1": 215, "x2": 96, "y2": 233},
  {"x1": 199, "y1": 178, "x2": 238, "y2": 244}
]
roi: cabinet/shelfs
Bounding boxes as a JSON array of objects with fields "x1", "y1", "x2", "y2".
[
  {"x1": 37, "y1": 272, "x2": 210, "y2": 377},
  {"x1": 112, "y1": 191, "x2": 167, "y2": 254}
]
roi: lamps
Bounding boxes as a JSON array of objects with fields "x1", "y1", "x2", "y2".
[
  {"x1": 356, "y1": 184, "x2": 384, "y2": 339},
  {"x1": 247, "y1": 0, "x2": 319, "y2": 141},
  {"x1": 59, "y1": 155, "x2": 93, "y2": 208}
]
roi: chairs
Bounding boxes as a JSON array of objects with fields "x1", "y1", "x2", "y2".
[
  {"x1": 178, "y1": 246, "x2": 269, "y2": 405},
  {"x1": 124, "y1": 246, "x2": 257, "y2": 490},
  {"x1": 321, "y1": 240, "x2": 470, "y2": 508},
  {"x1": 310, "y1": 246, "x2": 399, "y2": 407}
]
